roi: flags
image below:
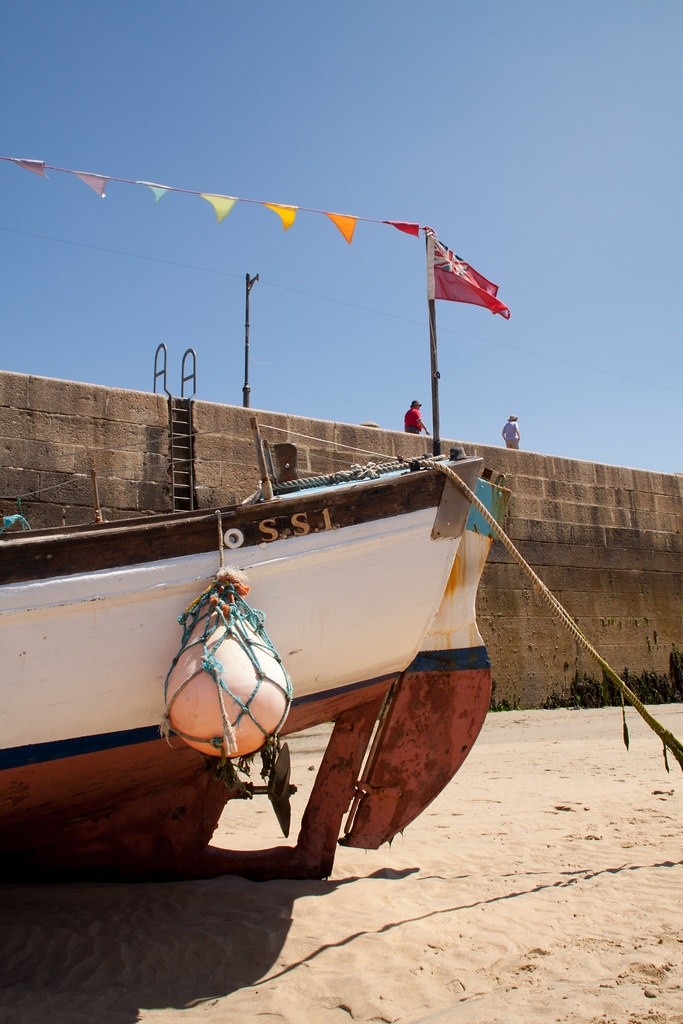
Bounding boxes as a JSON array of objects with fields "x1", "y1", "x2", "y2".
[{"x1": 427, "y1": 229, "x2": 513, "y2": 321}]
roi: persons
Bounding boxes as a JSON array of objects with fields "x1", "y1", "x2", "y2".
[
  {"x1": 502, "y1": 415, "x2": 522, "y2": 450},
  {"x1": 404, "y1": 400, "x2": 430, "y2": 435}
]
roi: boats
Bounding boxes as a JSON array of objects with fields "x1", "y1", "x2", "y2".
[{"x1": 0, "y1": 156, "x2": 512, "y2": 880}]
